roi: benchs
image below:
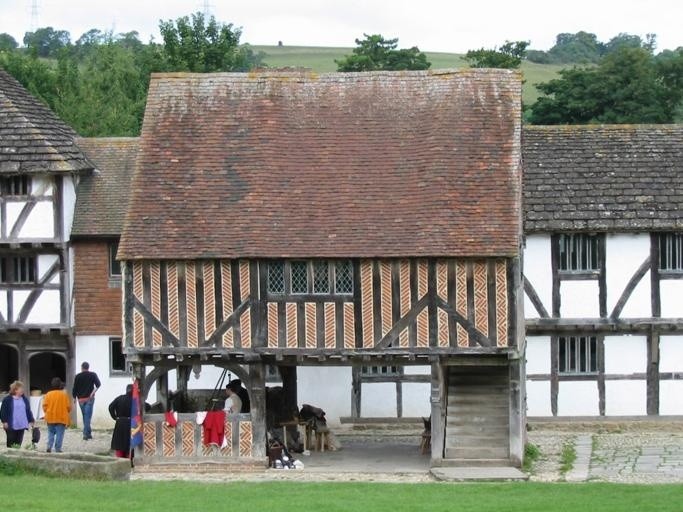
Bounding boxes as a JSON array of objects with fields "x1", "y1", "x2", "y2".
[
  {"x1": 415, "y1": 431, "x2": 430, "y2": 455},
  {"x1": 315, "y1": 418, "x2": 329, "y2": 452}
]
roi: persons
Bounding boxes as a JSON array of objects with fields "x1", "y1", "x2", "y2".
[
  {"x1": 73, "y1": 362, "x2": 101, "y2": 441},
  {"x1": 222, "y1": 378, "x2": 250, "y2": 414},
  {"x1": 42, "y1": 377, "x2": 72, "y2": 452},
  {"x1": 109, "y1": 384, "x2": 134, "y2": 458},
  {"x1": 0, "y1": 380, "x2": 34, "y2": 449}
]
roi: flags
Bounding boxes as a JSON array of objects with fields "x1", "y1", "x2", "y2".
[{"x1": 130, "y1": 381, "x2": 142, "y2": 449}]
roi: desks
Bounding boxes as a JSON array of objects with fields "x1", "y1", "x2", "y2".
[{"x1": 278, "y1": 415, "x2": 313, "y2": 453}]
